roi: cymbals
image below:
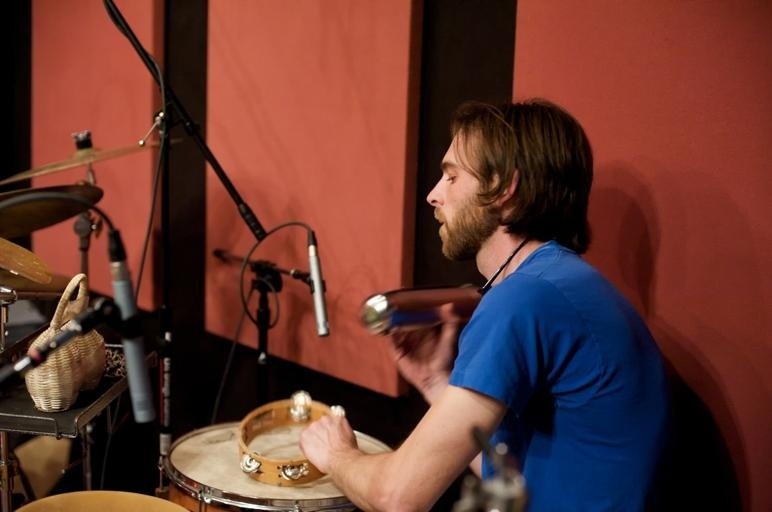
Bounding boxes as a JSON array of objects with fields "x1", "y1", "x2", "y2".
[
  {"x1": 0, "y1": 139, "x2": 183, "y2": 184},
  {"x1": 0, "y1": 185, "x2": 103, "y2": 237}
]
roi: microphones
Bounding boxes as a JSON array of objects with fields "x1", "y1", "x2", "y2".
[
  {"x1": 107, "y1": 231, "x2": 156, "y2": 424},
  {"x1": 308, "y1": 231, "x2": 330, "y2": 339}
]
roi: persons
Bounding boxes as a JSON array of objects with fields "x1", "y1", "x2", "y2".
[{"x1": 297, "y1": 99, "x2": 668, "y2": 512}]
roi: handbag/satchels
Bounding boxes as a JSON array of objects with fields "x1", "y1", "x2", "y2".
[{"x1": 25, "y1": 274, "x2": 106, "y2": 413}]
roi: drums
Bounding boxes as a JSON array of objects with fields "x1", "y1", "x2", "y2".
[{"x1": 165, "y1": 422, "x2": 395, "y2": 512}]
